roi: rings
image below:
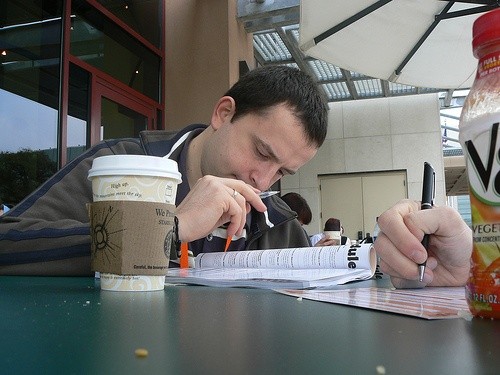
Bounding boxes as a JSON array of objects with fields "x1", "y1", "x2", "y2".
[{"x1": 233, "y1": 189, "x2": 237, "y2": 197}]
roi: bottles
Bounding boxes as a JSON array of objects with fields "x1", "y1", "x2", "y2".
[
  {"x1": 458, "y1": 10, "x2": 500, "y2": 321},
  {"x1": 372, "y1": 217, "x2": 381, "y2": 261}
]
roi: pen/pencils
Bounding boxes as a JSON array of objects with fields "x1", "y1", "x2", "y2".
[
  {"x1": 258, "y1": 190, "x2": 280, "y2": 199},
  {"x1": 417, "y1": 161, "x2": 436, "y2": 282}
]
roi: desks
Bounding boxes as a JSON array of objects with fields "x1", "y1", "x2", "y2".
[{"x1": 0, "y1": 272, "x2": 500, "y2": 375}]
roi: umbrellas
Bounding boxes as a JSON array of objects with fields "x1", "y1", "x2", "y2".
[{"x1": 300, "y1": 0, "x2": 500, "y2": 90}]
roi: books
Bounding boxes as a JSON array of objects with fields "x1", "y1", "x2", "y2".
[{"x1": 165, "y1": 244, "x2": 378, "y2": 289}]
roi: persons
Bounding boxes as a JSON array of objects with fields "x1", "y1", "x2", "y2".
[
  {"x1": 0, "y1": 65, "x2": 328, "y2": 276},
  {"x1": 280, "y1": 192, "x2": 337, "y2": 246},
  {"x1": 374, "y1": 199, "x2": 473, "y2": 289},
  {"x1": 324, "y1": 218, "x2": 350, "y2": 245}
]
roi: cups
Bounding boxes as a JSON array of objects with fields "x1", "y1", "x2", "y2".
[
  {"x1": 87, "y1": 154, "x2": 182, "y2": 292},
  {"x1": 323, "y1": 231, "x2": 341, "y2": 246}
]
